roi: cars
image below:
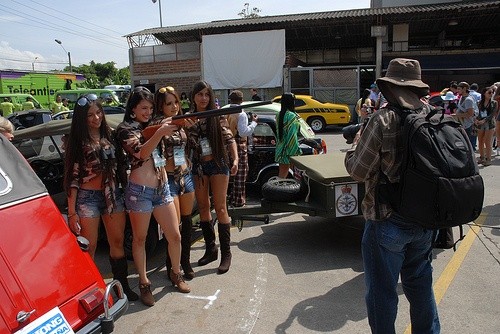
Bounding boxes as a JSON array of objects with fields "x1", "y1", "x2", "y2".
[
  {"x1": 0, "y1": 108, "x2": 126, "y2": 215},
  {"x1": 271, "y1": 94, "x2": 351, "y2": 133},
  {"x1": 420, "y1": 91, "x2": 446, "y2": 111},
  {"x1": 218, "y1": 101, "x2": 327, "y2": 199},
  {"x1": 0, "y1": 131, "x2": 129, "y2": 334}
]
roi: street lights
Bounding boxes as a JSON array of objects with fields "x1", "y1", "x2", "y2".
[
  {"x1": 55, "y1": 39, "x2": 72, "y2": 73},
  {"x1": 32, "y1": 56, "x2": 38, "y2": 72}
]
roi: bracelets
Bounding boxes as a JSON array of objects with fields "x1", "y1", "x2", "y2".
[{"x1": 68, "y1": 212, "x2": 77, "y2": 218}]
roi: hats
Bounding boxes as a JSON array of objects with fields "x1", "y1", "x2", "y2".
[
  {"x1": 456, "y1": 82, "x2": 470, "y2": 90},
  {"x1": 370, "y1": 84, "x2": 376, "y2": 88},
  {"x1": 441, "y1": 91, "x2": 457, "y2": 100},
  {"x1": 27, "y1": 96, "x2": 33, "y2": 99},
  {"x1": 229, "y1": 90, "x2": 243, "y2": 99},
  {"x1": 376, "y1": 58, "x2": 430, "y2": 104}
]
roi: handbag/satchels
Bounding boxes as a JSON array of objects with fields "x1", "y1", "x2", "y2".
[
  {"x1": 431, "y1": 226, "x2": 454, "y2": 249},
  {"x1": 474, "y1": 116, "x2": 481, "y2": 129}
]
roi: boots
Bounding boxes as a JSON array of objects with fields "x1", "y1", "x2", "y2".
[
  {"x1": 181, "y1": 215, "x2": 194, "y2": 278},
  {"x1": 109, "y1": 248, "x2": 139, "y2": 301},
  {"x1": 163, "y1": 233, "x2": 184, "y2": 282},
  {"x1": 198, "y1": 218, "x2": 218, "y2": 266},
  {"x1": 218, "y1": 223, "x2": 232, "y2": 273}
]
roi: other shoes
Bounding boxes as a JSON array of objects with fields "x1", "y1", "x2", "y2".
[
  {"x1": 483, "y1": 158, "x2": 491, "y2": 166},
  {"x1": 493, "y1": 146, "x2": 498, "y2": 150},
  {"x1": 493, "y1": 143, "x2": 495, "y2": 147},
  {"x1": 477, "y1": 157, "x2": 485, "y2": 164}
]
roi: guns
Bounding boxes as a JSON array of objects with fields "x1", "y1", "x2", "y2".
[{"x1": 139, "y1": 100, "x2": 273, "y2": 142}]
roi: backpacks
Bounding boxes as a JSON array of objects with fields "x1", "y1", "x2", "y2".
[{"x1": 379, "y1": 104, "x2": 484, "y2": 229}]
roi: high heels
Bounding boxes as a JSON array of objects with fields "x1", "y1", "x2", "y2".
[
  {"x1": 139, "y1": 279, "x2": 155, "y2": 306},
  {"x1": 170, "y1": 267, "x2": 191, "y2": 292}
]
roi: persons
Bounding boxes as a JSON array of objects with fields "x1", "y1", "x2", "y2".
[
  {"x1": 355, "y1": 82, "x2": 500, "y2": 167},
  {"x1": 344, "y1": 58, "x2": 442, "y2": 334},
  {"x1": 0, "y1": 80, "x2": 302, "y2": 306}
]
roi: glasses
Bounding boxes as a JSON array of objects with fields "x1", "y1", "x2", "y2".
[
  {"x1": 159, "y1": 86, "x2": 174, "y2": 93},
  {"x1": 489, "y1": 91, "x2": 494, "y2": 94},
  {"x1": 131, "y1": 87, "x2": 151, "y2": 94},
  {"x1": 78, "y1": 94, "x2": 97, "y2": 107}
]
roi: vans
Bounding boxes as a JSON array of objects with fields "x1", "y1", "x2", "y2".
[
  {"x1": 0, "y1": 93, "x2": 44, "y2": 117},
  {"x1": 103, "y1": 84, "x2": 131, "y2": 103},
  {"x1": 52, "y1": 88, "x2": 121, "y2": 108}
]
